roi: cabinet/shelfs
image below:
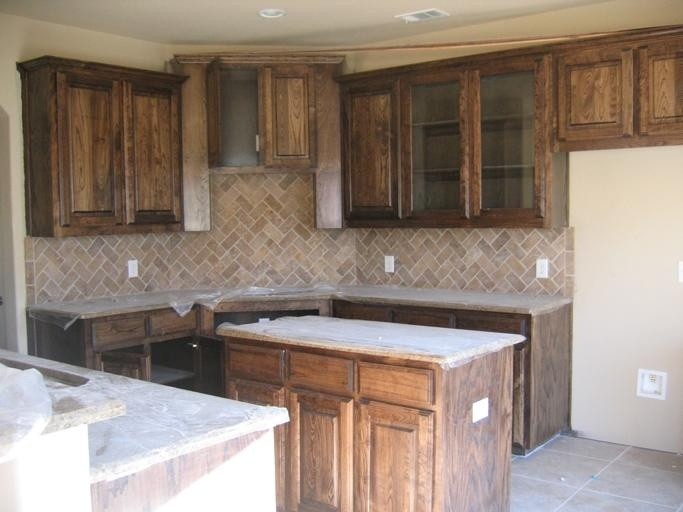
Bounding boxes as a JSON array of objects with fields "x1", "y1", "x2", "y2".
[{"x1": 214, "y1": 316, "x2": 526, "y2": 512}]
